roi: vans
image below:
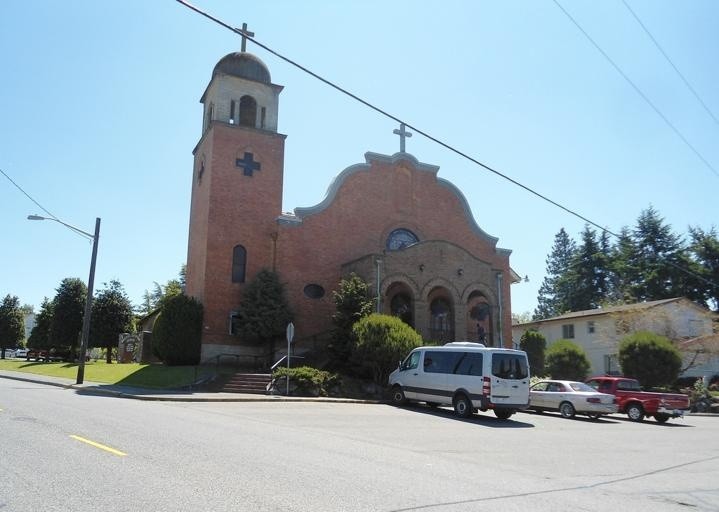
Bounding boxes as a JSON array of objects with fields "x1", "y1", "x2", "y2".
[{"x1": 387, "y1": 341, "x2": 531, "y2": 419}]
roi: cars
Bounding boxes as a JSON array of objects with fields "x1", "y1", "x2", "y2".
[
  {"x1": 517, "y1": 379, "x2": 620, "y2": 419},
  {"x1": 0, "y1": 348, "x2": 91, "y2": 362}
]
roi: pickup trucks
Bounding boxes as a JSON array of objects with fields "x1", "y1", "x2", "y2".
[{"x1": 580, "y1": 376, "x2": 691, "y2": 423}]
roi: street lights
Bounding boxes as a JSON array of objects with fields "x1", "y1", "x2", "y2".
[{"x1": 27, "y1": 214, "x2": 102, "y2": 385}]
